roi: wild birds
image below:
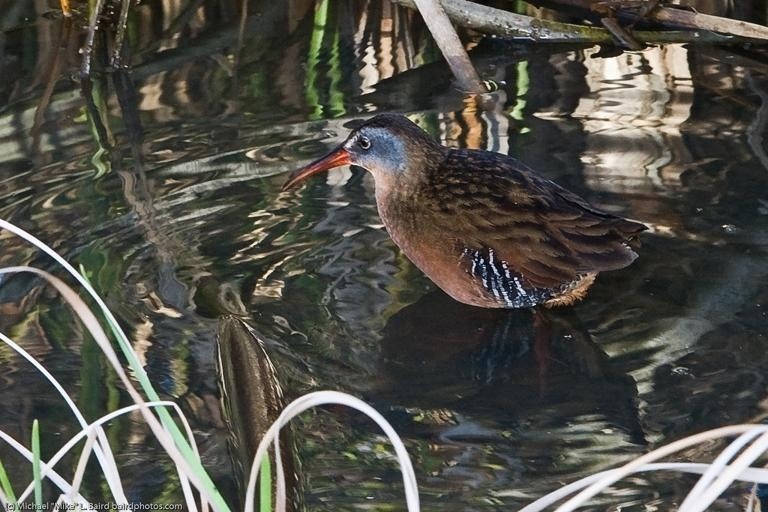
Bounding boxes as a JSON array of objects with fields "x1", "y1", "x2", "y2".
[{"x1": 279, "y1": 111, "x2": 650, "y2": 399}]
